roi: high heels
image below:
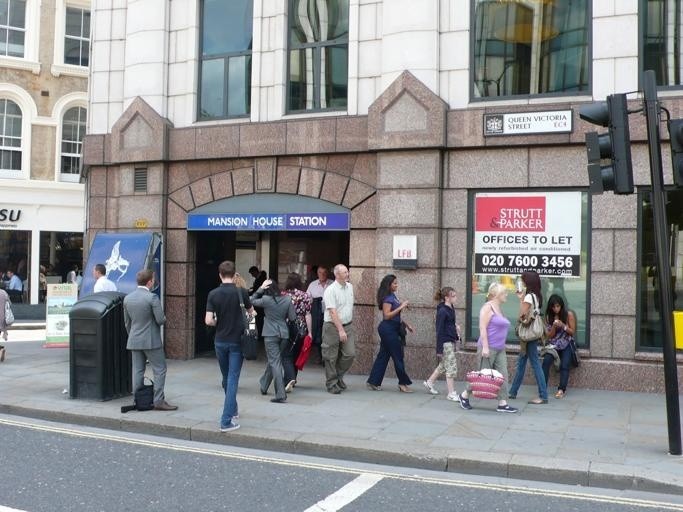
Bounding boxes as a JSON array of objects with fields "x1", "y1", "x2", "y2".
[
  {"x1": 397, "y1": 383, "x2": 413, "y2": 393},
  {"x1": 367, "y1": 381, "x2": 383, "y2": 390}
]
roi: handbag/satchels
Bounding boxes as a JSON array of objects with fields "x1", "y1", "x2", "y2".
[
  {"x1": 517, "y1": 293, "x2": 544, "y2": 342},
  {"x1": 455, "y1": 324, "x2": 463, "y2": 352},
  {"x1": 571, "y1": 350, "x2": 581, "y2": 368},
  {"x1": 466, "y1": 357, "x2": 505, "y2": 400},
  {"x1": 134, "y1": 376, "x2": 164, "y2": 411},
  {"x1": 294, "y1": 336, "x2": 312, "y2": 371},
  {"x1": 239, "y1": 328, "x2": 258, "y2": 360},
  {"x1": 1, "y1": 290, "x2": 14, "y2": 325}
]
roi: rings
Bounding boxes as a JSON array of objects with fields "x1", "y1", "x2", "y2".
[{"x1": 405, "y1": 303, "x2": 407, "y2": 305}]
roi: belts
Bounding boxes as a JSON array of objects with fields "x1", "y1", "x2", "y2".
[{"x1": 342, "y1": 321, "x2": 353, "y2": 327}]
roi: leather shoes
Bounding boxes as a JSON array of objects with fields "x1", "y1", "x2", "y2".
[{"x1": 152, "y1": 399, "x2": 177, "y2": 410}]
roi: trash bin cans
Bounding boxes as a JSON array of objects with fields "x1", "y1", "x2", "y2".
[{"x1": 70, "y1": 291, "x2": 133, "y2": 402}]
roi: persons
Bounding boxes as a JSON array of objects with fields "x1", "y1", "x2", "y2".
[
  {"x1": 367, "y1": 274, "x2": 414, "y2": 394},
  {"x1": 0, "y1": 264, "x2": 59, "y2": 362},
  {"x1": 458, "y1": 283, "x2": 519, "y2": 413},
  {"x1": 204, "y1": 256, "x2": 357, "y2": 433},
  {"x1": 93, "y1": 262, "x2": 118, "y2": 292},
  {"x1": 122, "y1": 269, "x2": 178, "y2": 411},
  {"x1": 542, "y1": 294, "x2": 578, "y2": 398},
  {"x1": 423, "y1": 287, "x2": 460, "y2": 402},
  {"x1": 66, "y1": 263, "x2": 79, "y2": 284},
  {"x1": 508, "y1": 270, "x2": 549, "y2": 405}
]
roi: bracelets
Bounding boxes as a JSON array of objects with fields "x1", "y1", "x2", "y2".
[{"x1": 563, "y1": 326, "x2": 567, "y2": 332}]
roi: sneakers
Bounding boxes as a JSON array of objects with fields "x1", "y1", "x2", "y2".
[
  {"x1": 220, "y1": 422, "x2": 240, "y2": 433},
  {"x1": 326, "y1": 384, "x2": 342, "y2": 394},
  {"x1": 231, "y1": 405, "x2": 239, "y2": 419},
  {"x1": 423, "y1": 380, "x2": 439, "y2": 395},
  {"x1": 555, "y1": 390, "x2": 567, "y2": 399},
  {"x1": 259, "y1": 381, "x2": 267, "y2": 394},
  {"x1": 337, "y1": 380, "x2": 347, "y2": 389},
  {"x1": 447, "y1": 391, "x2": 460, "y2": 401},
  {"x1": 496, "y1": 404, "x2": 518, "y2": 413},
  {"x1": 270, "y1": 395, "x2": 287, "y2": 402},
  {"x1": 459, "y1": 393, "x2": 472, "y2": 410},
  {"x1": 528, "y1": 398, "x2": 548, "y2": 404},
  {"x1": 285, "y1": 378, "x2": 296, "y2": 393},
  {"x1": 508, "y1": 394, "x2": 516, "y2": 399}
]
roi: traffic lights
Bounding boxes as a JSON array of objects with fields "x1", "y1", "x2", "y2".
[
  {"x1": 665, "y1": 117, "x2": 683, "y2": 189},
  {"x1": 574, "y1": 87, "x2": 635, "y2": 201}
]
